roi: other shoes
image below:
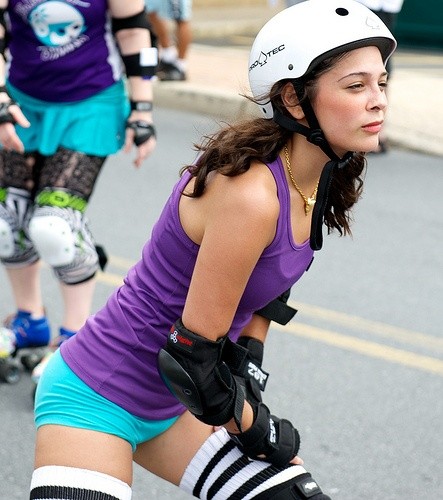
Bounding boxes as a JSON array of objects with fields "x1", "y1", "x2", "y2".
[{"x1": 156, "y1": 61, "x2": 186, "y2": 81}]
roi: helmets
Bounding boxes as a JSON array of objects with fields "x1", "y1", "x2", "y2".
[{"x1": 250, "y1": 0, "x2": 397, "y2": 120}]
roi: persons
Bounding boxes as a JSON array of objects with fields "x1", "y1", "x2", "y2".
[
  {"x1": 28, "y1": 0, "x2": 397, "y2": 500},
  {"x1": 143, "y1": 0, "x2": 193, "y2": 83},
  {"x1": 0, "y1": 0, "x2": 157, "y2": 385}
]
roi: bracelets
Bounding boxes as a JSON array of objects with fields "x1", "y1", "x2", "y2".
[{"x1": 129, "y1": 101, "x2": 153, "y2": 111}]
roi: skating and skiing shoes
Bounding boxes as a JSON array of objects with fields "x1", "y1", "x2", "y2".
[
  {"x1": 31, "y1": 327, "x2": 81, "y2": 406},
  {"x1": 0, "y1": 310, "x2": 51, "y2": 386}
]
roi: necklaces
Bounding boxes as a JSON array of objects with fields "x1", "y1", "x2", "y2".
[{"x1": 283, "y1": 142, "x2": 321, "y2": 217}]
